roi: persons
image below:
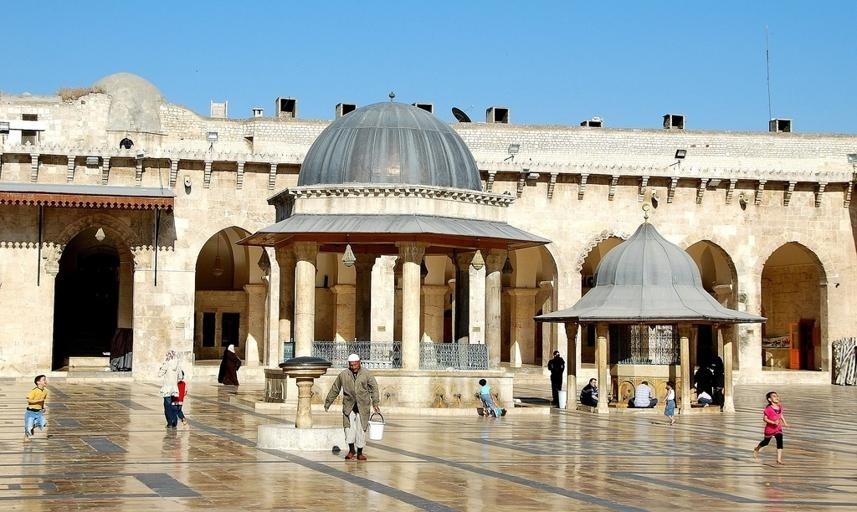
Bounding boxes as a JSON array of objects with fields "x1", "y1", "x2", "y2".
[
  {"x1": 580, "y1": 378, "x2": 598, "y2": 407},
  {"x1": 479, "y1": 379, "x2": 499, "y2": 418},
  {"x1": 628, "y1": 381, "x2": 658, "y2": 409},
  {"x1": 548, "y1": 351, "x2": 565, "y2": 408},
  {"x1": 697, "y1": 388, "x2": 712, "y2": 407},
  {"x1": 753, "y1": 392, "x2": 788, "y2": 464},
  {"x1": 662, "y1": 381, "x2": 676, "y2": 425},
  {"x1": 691, "y1": 361, "x2": 712, "y2": 407},
  {"x1": 324, "y1": 353, "x2": 380, "y2": 460},
  {"x1": 158, "y1": 349, "x2": 182, "y2": 428},
  {"x1": 712, "y1": 357, "x2": 724, "y2": 404},
  {"x1": 24, "y1": 375, "x2": 49, "y2": 442},
  {"x1": 172, "y1": 370, "x2": 188, "y2": 424},
  {"x1": 218, "y1": 344, "x2": 241, "y2": 385}
]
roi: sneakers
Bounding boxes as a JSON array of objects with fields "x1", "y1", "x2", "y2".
[
  {"x1": 182, "y1": 418, "x2": 188, "y2": 428},
  {"x1": 357, "y1": 453, "x2": 367, "y2": 461},
  {"x1": 345, "y1": 449, "x2": 356, "y2": 459}
]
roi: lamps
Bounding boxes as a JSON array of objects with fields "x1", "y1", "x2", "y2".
[
  {"x1": 95, "y1": 208, "x2": 108, "y2": 242},
  {"x1": 339, "y1": 235, "x2": 356, "y2": 267},
  {"x1": 211, "y1": 232, "x2": 223, "y2": 277},
  {"x1": 504, "y1": 143, "x2": 519, "y2": 163},
  {"x1": 0, "y1": 121, "x2": 11, "y2": 145},
  {"x1": 469, "y1": 237, "x2": 485, "y2": 272},
  {"x1": 204, "y1": 133, "x2": 220, "y2": 151},
  {"x1": 668, "y1": 150, "x2": 687, "y2": 168},
  {"x1": 256, "y1": 246, "x2": 270, "y2": 271}
]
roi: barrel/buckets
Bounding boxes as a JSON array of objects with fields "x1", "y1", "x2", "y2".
[
  {"x1": 558, "y1": 389, "x2": 567, "y2": 408},
  {"x1": 367, "y1": 411, "x2": 386, "y2": 439}
]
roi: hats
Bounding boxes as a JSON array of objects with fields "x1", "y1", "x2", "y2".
[{"x1": 347, "y1": 353, "x2": 360, "y2": 362}]
